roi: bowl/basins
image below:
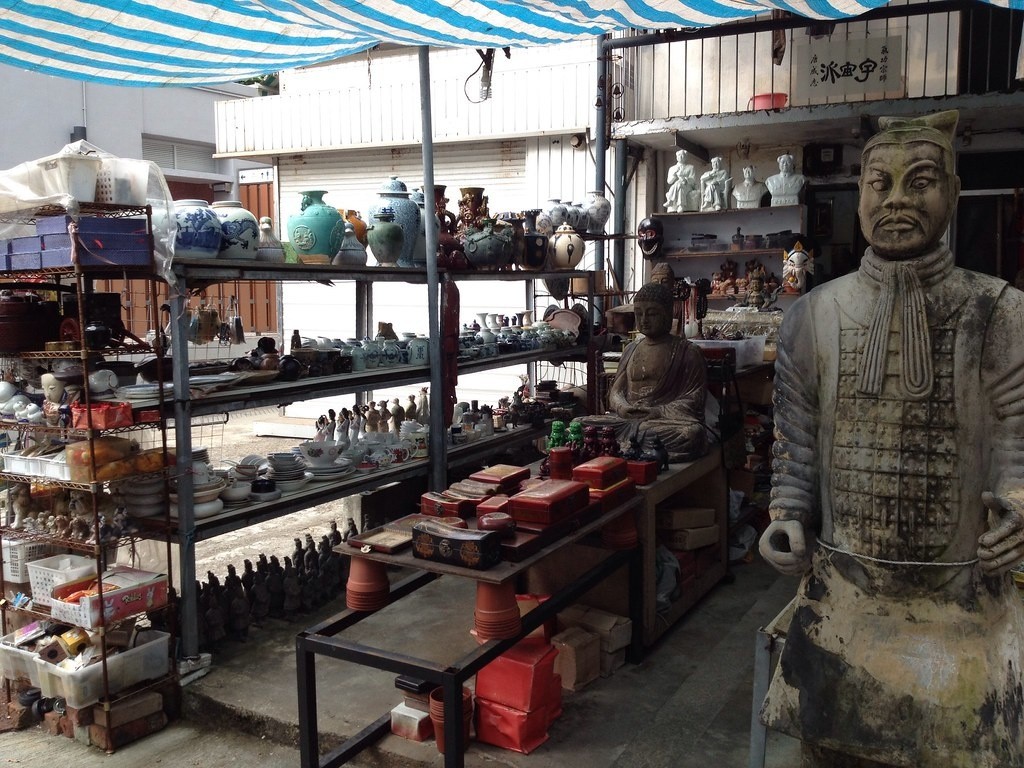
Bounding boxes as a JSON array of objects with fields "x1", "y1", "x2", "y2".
[
  {"x1": 299, "y1": 441, "x2": 346, "y2": 466},
  {"x1": 221, "y1": 482, "x2": 251, "y2": 503}
]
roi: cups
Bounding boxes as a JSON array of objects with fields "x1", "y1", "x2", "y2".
[{"x1": 365, "y1": 440, "x2": 418, "y2": 467}]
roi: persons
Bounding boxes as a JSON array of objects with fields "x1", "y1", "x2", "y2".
[
  {"x1": 565, "y1": 282, "x2": 710, "y2": 462},
  {"x1": 734, "y1": 277, "x2": 771, "y2": 309},
  {"x1": 752, "y1": 108, "x2": 1024, "y2": 768},
  {"x1": 86, "y1": 505, "x2": 129, "y2": 544},
  {"x1": 193, "y1": 513, "x2": 380, "y2": 651},
  {"x1": 313, "y1": 385, "x2": 429, "y2": 451},
  {"x1": 649, "y1": 261, "x2": 674, "y2": 285},
  {"x1": 0, "y1": 372, "x2": 83, "y2": 444},
  {"x1": 661, "y1": 148, "x2": 805, "y2": 214}
]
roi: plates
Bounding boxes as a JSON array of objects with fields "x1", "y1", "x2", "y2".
[
  {"x1": 121, "y1": 383, "x2": 174, "y2": 403},
  {"x1": 264, "y1": 441, "x2": 357, "y2": 491},
  {"x1": 192, "y1": 446, "x2": 210, "y2": 464},
  {"x1": 190, "y1": 374, "x2": 240, "y2": 388},
  {"x1": 123, "y1": 468, "x2": 225, "y2": 518}
]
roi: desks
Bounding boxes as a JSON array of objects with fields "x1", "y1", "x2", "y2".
[{"x1": 523, "y1": 440, "x2": 729, "y2": 663}]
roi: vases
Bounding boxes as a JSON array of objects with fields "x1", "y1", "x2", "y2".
[
  {"x1": 603, "y1": 510, "x2": 637, "y2": 549},
  {"x1": 474, "y1": 581, "x2": 522, "y2": 640},
  {"x1": 430, "y1": 684, "x2": 472, "y2": 754},
  {"x1": 346, "y1": 556, "x2": 390, "y2": 610}
]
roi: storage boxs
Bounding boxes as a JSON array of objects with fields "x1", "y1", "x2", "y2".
[
  {"x1": 589, "y1": 477, "x2": 636, "y2": 513},
  {"x1": 1, "y1": 539, "x2": 169, "y2": 628},
  {"x1": 626, "y1": 460, "x2": 657, "y2": 486},
  {"x1": 692, "y1": 335, "x2": 767, "y2": 367},
  {"x1": 1, "y1": 450, "x2": 71, "y2": 481},
  {"x1": 571, "y1": 457, "x2": 626, "y2": 489},
  {"x1": 73, "y1": 402, "x2": 133, "y2": 429},
  {"x1": 0, "y1": 292, "x2": 121, "y2": 352},
  {"x1": 390, "y1": 674, "x2": 435, "y2": 742},
  {"x1": 0, "y1": 620, "x2": 171, "y2": 710},
  {"x1": 0, "y1": 154, "x2": 151, "y2": 271},
  {"x1": 659, "y1": 509, "x2": 720, "y2": 586},
  {"x1": 346, "y1": 456, "x2": 599, "y2": 570},
  {"x1": 471, "y1": 591, "x2": 632, "y2": 755}
]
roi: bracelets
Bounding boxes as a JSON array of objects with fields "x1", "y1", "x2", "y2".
[
  {"x1": 676, "y1": 280, "x2": 691, "y2": 300},
  {"x1": 695, "y1": 278, "x2": 712, "y2": 341}
]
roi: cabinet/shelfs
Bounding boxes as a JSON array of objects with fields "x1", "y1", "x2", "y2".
[
  {"x1": 651, "y1": 204, "x2": 805, "y2": 299},
  {"x1": 0, "y1": 203, "x2": 178, "y2": 756},
  {"x1": 83, "y1": 258, "x2": 596, "y2": 671},
  {"x1": 596, "y1": 359, "x2": 775, "y2": 582}
]
